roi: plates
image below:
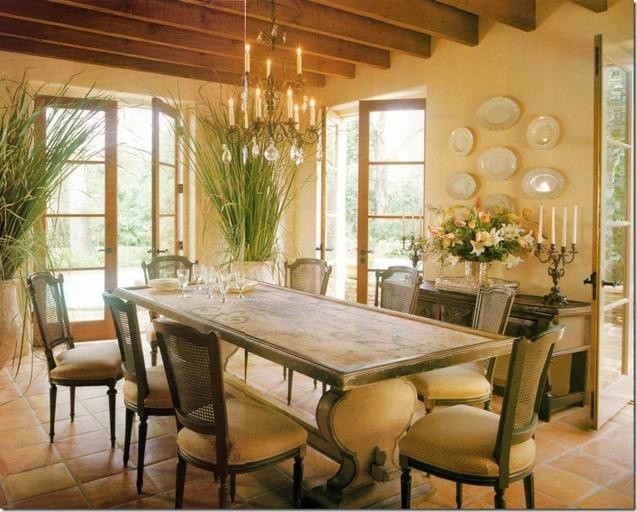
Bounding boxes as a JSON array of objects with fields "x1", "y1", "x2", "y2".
[
  {"x1": 445, "y1": 172, "x2": 477, "y2": 201},
  {"x1": 448, "y1": 127, "x2": 474, "y2": 158},
  {"x1": 475, "y1": 145, "x2": 518, "y2": 182},
  {"x1": 522, "y1": 167, "x2": 567, "y2": 201},
  {"x1": 526, "y1": 115, "x2": 561, "y2": 152},
  {"x1": 473, "y1": 97, "x2": 520, "y2": 132}
]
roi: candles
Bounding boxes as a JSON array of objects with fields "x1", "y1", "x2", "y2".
[
  {"x1": 561, "y1": 205, "x2": 568, "y2": 247},
  {"x1": 551, "y1": 206, "x2": 556, "y2": 246},
  {"x1": 411, "y1": 206, "x2": 414, "y2": 238},
  {"x1": 572, "y1": 203, "x2": 578, "y2": 245},
  {"x1": 419, "y1": 208, "x2": 423, "y2": 239},
  {"x1": 402, "y1": 210, "x2": 406, "y2": 238},
  {"x1": 537, "y1": 203, "x2": 544, "y2": 245}
]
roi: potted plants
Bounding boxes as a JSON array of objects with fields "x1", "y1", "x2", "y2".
[
  {"x1": 155, "y1": 80, "x2": 326, "y2": 285},
  {"x1": 1, "y1": 64, "x2": 151, "y2": 398}
]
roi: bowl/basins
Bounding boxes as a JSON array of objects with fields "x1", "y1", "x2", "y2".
[{"x1": 149, "y1": 277, "x2": 178, "y2": 291}]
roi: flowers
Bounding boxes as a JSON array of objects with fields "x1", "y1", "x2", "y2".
[{"x1": 414, "y1": 196, "x2": 537, "y2": 270}]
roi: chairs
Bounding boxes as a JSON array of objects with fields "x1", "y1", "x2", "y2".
[
  {"x1": 141, "y1": 255, "x2": 198, "y2": 367},
  {"x1": 152, "y1": 319, "x2": 308, "y2": 510},
  {"x1": 401, "y1": 281, "x2": 514, "y2": 414},
  {"x1": 101, "y1": 289, "x2": 174, "y2": 495},
  {"x1": 243, "y1": 257, "x2": 333, "y2": 407},
  {"x1": 28, "y1": 271, "x2": 124, "y2": 448},
  {"x1": 319, "y1": 266, "x2": 423, "y2": 396},
  {"x1": 397, "y1": 321, "x2": 566, "y2": 510}
]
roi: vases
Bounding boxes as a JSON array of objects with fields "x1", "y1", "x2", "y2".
[{"x1": 464, "y1": 261, "x2": 487, "y2": 288}]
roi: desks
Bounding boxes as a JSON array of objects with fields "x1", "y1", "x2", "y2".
[{"x1": 377, "y1": 279, "x2": 591, "y2": 422}]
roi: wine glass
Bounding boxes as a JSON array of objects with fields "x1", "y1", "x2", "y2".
[
  {"x1": 176, "y1": 268, "x2": 190, "y2": 300},
  {"x1": 192, "y1": 263, "x2": 248, "y2": 304}
]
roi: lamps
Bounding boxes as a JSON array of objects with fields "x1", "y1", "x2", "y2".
[{"x1": 222, "y1": 1, "x2": 319, "y2": 168}]
